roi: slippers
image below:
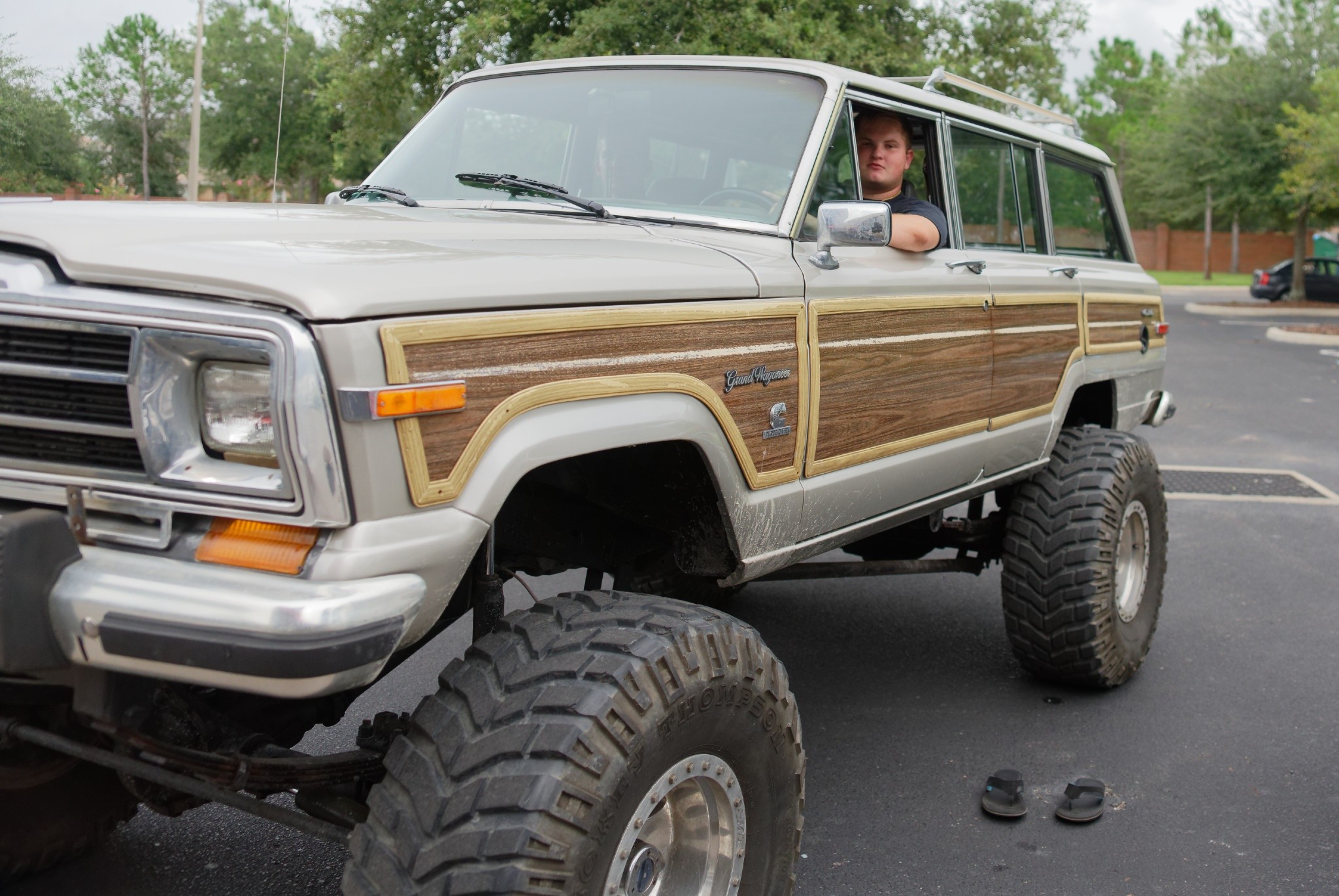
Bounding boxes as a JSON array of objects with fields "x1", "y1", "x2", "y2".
[
  {"x1": 982, "y1": 768, "x2": 1028, "y2": 815},
  {"x1": 1054, "y1": 778, "x2": 1105, "y2": 822}
]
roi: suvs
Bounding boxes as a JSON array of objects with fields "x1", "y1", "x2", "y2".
[{"x1": 0, "y1": 54, "x2": 1180, "y2": 896}]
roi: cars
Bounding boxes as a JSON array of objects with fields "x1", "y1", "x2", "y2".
[{"x1": 1249, "y1": 254, "x2": 1338, "y2": 304}]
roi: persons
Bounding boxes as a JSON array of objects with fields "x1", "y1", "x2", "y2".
[{"x1": 762, "y1": 107, "x2": 949, "y2": 254}]
